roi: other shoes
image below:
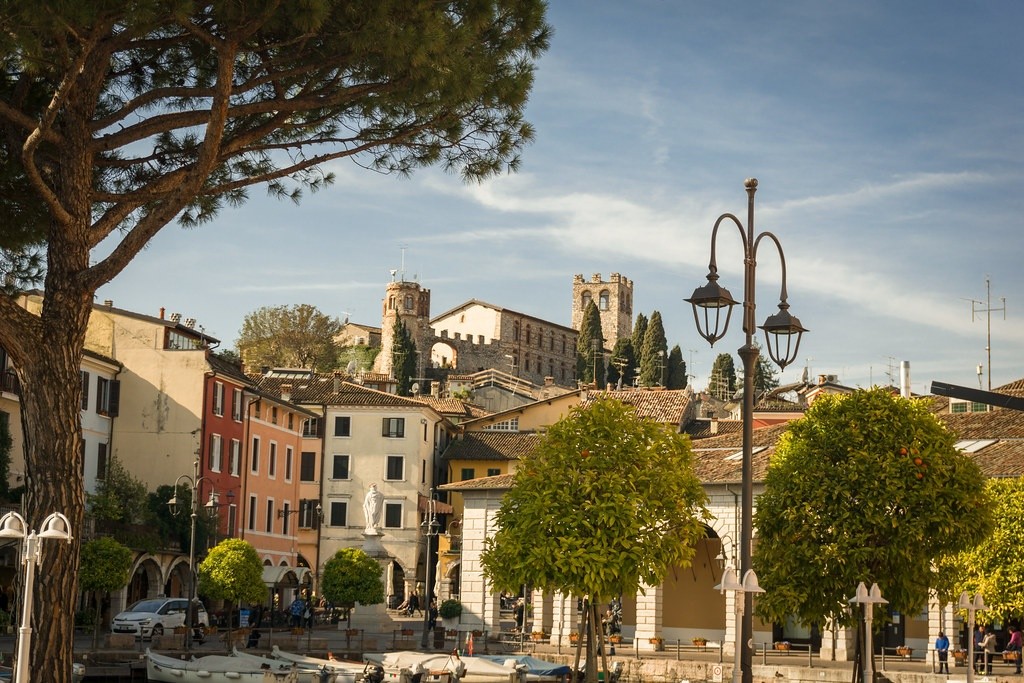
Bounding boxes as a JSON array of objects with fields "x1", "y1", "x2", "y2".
[
  {"x1": 947, "y1": 672, "x2": 951, "y2": 673},
  {"x1": 936, "y1": 671, "x2": 942, "y2": 674},
  {"x1": 607, "y1": 653, "x2": 615, "y2": 656},
  {"x1": 199, "y1": 640, "x2": 206, "y2": 646},
  {"x1": 1014, "y1": 670, "x2": 1021, "y2": 674}
]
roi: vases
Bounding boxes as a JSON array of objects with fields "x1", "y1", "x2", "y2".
[
  {"x1": 775, "y1": 643, "x2": 791, "y2": 650},
  {"x1": 1003, "y1": 651, "x2": 1020, "y2": 660},
  {"x1": 175, "y1": 626, "x2": 187, "y2": 634},
  {"x1": 570, "y1": 635, "x2": 580, "y2": 642},
  {"x1": 446, "y1": 629, "x2": 458, "y2": 636},
  {"x1": 954, "y1": 652, "x2": 968, "y2": 660},
  {"x1": 473, "y1": 631, "x2": 482, "y2": 637},
  {"x1": 896, "y1": 649, "x2": 913, "y2": 656}
]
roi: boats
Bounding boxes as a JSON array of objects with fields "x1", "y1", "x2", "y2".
[{"x1": 0, "y1": 623, "x2": 625, "y2": 683}]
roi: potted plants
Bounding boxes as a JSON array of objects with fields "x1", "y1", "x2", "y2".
[
  {"x1": 532, "y1": 631, "x2": 545, "y2": 639},
  {"x1": 649, "y1": 636, "x2": 665, "y2": 645},
  {"x1": 610, "y1": 634, "x2": 624, "y2": 643},
  {"x1": 402, "y1": 628, "x2": 413, "y2": 636},
  {"x1": 203, "y1": 627, "x2": 217, "y2": 636},
  {"x1": 291, "y1": 628, "x2": 304, "y2": 635},
  {"x1": 346, "y1": 628, "x2": 358, "y2": 636},
  {"x1": 439, "y1": 600, "x2": 463, "y2": 632},
  {"x1": 692, "y1": 637, "x2": 706, "y2": 646}
]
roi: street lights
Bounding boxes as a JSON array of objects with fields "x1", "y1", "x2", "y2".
[
  {"x1": 165, "y1": 461, "x2": 224, "y2": 651},
  {"x1": 419, "y1": 486, "x2": 442, "y2": 653},
  {"x1": 958, "y1": 591, "x2": 989, "y2": 683},
  {"x1": 0, "y1": 511, "x2": 75, "y2": 683},
  {"x1": 847, "y1": 580, "x2": 890, "y2": 683},
  {"x1": 684, "y1": 175, "x2": 810, "y2": 683},
  {"x1": 712, "y1": 566, "x2": 766, "y2": 683}
]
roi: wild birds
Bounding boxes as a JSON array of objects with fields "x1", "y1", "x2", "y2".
[{"x1": 775, "y1": 670, "x2": 783, "y2": 678}]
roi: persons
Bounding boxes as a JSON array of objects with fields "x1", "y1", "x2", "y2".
[
  {"x1": 974, "y1": 625, "x2": 1024, "y2": 674},
  {"x1": 289, "y1": 595, "x2": 315, "y2": 633},
  {"x1": 935, "y1": 631, "x2": 950, "y2": 674},
  {"x1": 597, "y1": 610, "x2": 616, "y2": 656},
  {"x1": 427, "y1": 602, "x2": 438, "y2": 632},
  {"x1": 363, "y1": 483, "x2": 384, "y2": 528},
  {"x1": 406, "y1": 591, "x2": 423, "y2": 618},
  {"x1": 246, "y1": 600, "x2": 262, "y2": 649},
  {"x1": 183, "y1": 597, "x2": 208, "y2": 645},
  {"x1": 513, "y1": 600, "x2": 524, "y2": 631}
]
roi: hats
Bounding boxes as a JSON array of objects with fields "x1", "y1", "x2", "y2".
[{"x1": 606, "y1": 604, "x2": 612, "y2": 610}]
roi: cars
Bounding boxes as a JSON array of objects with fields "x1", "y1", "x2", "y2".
[{"x1": 110, "y1": 593, "x2": 210, "y2": 646}]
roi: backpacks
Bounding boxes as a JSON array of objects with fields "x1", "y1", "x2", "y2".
[{"x1": 303, "y1": 606, "x2": 312, "y2": 619}]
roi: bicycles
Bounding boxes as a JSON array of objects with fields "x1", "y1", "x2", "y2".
[{"x1": 502, "y1": 623, "x2": 536, "y2": 654}]
